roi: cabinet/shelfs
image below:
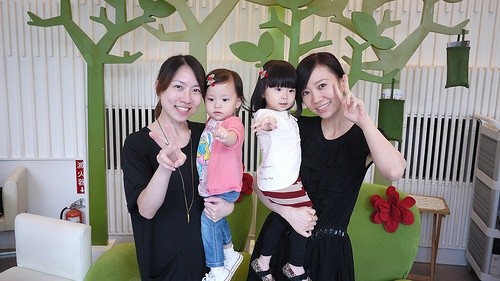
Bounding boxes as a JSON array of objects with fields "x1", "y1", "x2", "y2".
[{"x1": 466, "y1": 113, "x2": 500, "y2": 281}]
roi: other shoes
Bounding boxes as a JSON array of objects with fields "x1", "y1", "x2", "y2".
[
  {"x1": 250, "y1": 258, "x2": 276, "y2": 281},
  {"x1": 283, "y1": 263, "x2": 312, "y2": 281}
]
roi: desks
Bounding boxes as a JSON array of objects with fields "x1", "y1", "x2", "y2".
[{"x1": 408, "y1": 194, "x2": 449, "y2": 281}]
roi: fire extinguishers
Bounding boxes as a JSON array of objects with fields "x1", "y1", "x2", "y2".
[{"x1": 59, "y1": 197, "x2": 85, "y2": 225}]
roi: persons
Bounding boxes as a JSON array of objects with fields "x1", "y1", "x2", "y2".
[
  {"x1": 120, "y1": 55, "x2": 234, "y2": 281},
  {"x1": 196, "y1": 68, "x2": 247, "y2": 281},
  {"x1": 247, "y1": 52, "x2": 406, "y2": 281}
]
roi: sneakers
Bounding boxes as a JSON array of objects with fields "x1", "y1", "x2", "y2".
[{"x1": 202, "y1": 251, "x2": 244, "y2": 280}]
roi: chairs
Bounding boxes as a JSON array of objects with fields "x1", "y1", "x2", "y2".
[
  {"x1": 255, "y1": 182, "x2": 420, "y2": 281},
  {"x1": 0, "y1": 213, "x2": 92, "y2": 281},
  {"x1": 84, "y1": 165, "x2": 254, "y2": 281},
  {"x1": 0, "y1": 167, "x2": 27, "y2": 233}
]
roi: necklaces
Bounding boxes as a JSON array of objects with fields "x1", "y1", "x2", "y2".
[{"x1": 155, "y1": 118, "x2": 195, "y2": 225}]
